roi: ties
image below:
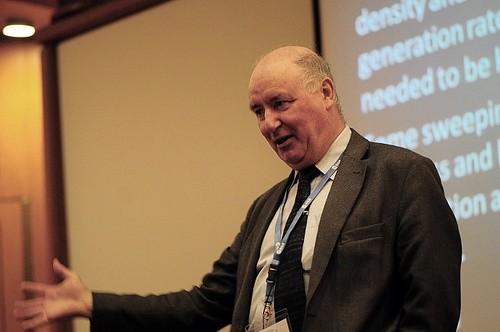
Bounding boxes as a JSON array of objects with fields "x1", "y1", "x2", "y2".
[{"x1": 273, "y1": 165, "x2": 322, "y2": 332}]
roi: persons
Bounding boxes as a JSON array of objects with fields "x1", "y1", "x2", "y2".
[{"x1": 15, "y1": 44, "x2": 464, "y2": 332}]
